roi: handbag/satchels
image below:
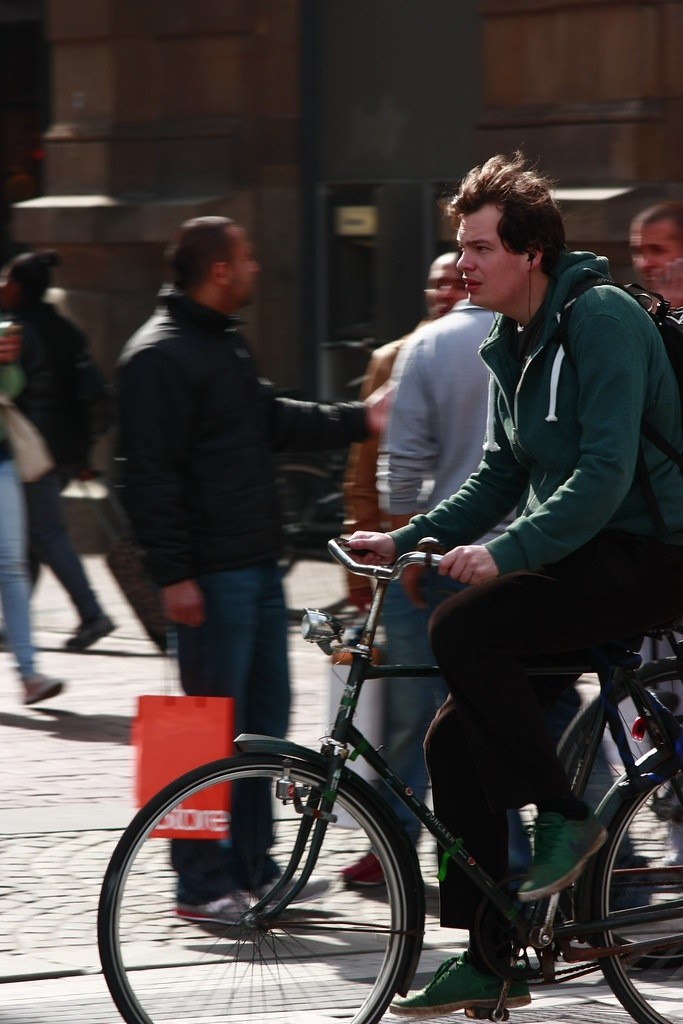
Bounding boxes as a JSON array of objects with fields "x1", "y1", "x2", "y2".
[
  {"x1": 0, "y1": 395, "x2": 55, "y2": 483},
  {"x1": 134, "y1": 624, "x2": 235, "y2": 839}
]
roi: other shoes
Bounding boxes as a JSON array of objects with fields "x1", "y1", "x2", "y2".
[
  {"x1": 65, "y1": 614, "x2": 114, "y2": 652},
  {"x1": 23, "y1": 673, "x2": 64, "y2": 706}
]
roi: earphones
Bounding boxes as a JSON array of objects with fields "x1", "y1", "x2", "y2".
[{"x1": 527, "y1": 253, "x2": 535, "y2": 262}]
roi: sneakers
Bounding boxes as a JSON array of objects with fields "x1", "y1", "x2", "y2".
[
  {"x1": 516, "y1": 802, "x2": 608, "y2": 903},
  {"x1": 341, "y1": 853, "x2": 385, "y2": 886},
  {"x1": 251, "y1": 874, "x2": 331, "y2": 904},
  {"x1": 177, "y1": 892, "x2": 254, "y2": 925},
  {"x1": 390, "y1": 950, "x2": 531, "y2": 1016}
]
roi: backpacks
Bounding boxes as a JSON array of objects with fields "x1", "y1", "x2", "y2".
[{"x1": 559, "y1": 278, "x2": 683, "y2": 470}]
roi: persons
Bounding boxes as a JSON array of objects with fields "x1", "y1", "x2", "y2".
[
  {"x1": 629, "y1": 199, "x2": 683, "y2": 815},
  {"x1": 346, "y1": 252, "x2": 470, "y2": 604},
  {"x1": 0, "y1": 237, "x2": 64, "y2": 705},
  {"x1": 108, "y1": 214, "x2": 394, "y2": 926},
  {"x1": 340, "y1": 252, "x2": 502, "y2": 886},
  {"x1": 344, "y1": 156, "x2": 683, "y2": 1014},
  {"x1": 0, "y1": 253, "x2": 115, "y2": 654}
]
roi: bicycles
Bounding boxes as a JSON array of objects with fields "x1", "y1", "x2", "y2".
[
  {"x1": 279, "y1": 341, "x2": 378, "y2": 556},
  {"x1": 96, "y1": 534, "x2": 683, "y2": 1023}
]
